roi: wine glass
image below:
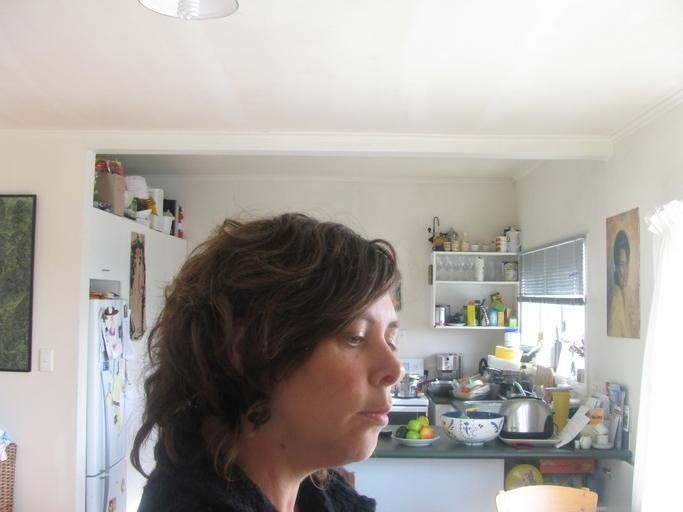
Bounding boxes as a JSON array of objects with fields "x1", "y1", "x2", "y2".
[{"x1": 436, "y1": 255, "x2": 474, "y2": 281}]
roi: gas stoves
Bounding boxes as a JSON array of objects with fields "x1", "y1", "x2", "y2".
[{"x1": 389, "y1": 391, "x2": 429, "y2": 407}]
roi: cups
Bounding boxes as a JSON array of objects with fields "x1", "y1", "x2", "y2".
[
  {"x1": 487, "y1": 256, "x2": 497, "y2": 281},
  {"x1": 579, "y1": 434, "x2": 592, "y2": 449},
  {"x1": 596, "y1": 434, "x2": 608, "y2": 445},
  {"x1": 443, "y1": 234, "x2": 507, "y2": 252},
  {"x1": 552, "y1": 391, "x2": 572, "y2": 434},
  {"x1": 475, "y1": 256, "x2": 485, "y2": 281}
]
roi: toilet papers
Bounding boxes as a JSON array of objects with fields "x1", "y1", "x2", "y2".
[{"x1": 475, "y1": 256, "x2": 484, "y2": 282}]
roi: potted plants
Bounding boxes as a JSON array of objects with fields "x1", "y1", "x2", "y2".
[{"x1": 569, "y1": 338, "x2": 585, "y2": 383}]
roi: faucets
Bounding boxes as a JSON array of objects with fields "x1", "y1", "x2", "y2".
[{"x1": 512, "y1": 380, "x2": 538, "y2": 399}]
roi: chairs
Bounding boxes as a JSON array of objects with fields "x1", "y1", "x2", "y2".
[{"x1": 495, "y1": 486, "x2": 599, "y2": 512}]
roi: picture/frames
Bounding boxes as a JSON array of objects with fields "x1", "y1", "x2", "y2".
[{"x1": 0, "y1": 195, "x2": 37, "y2": 372}]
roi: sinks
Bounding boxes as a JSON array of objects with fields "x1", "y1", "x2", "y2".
[{"x1": 450, "y1": 399, "x2": 508, "y2": 414}]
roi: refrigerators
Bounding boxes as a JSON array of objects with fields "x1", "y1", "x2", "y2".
[{"x1": 84, "y1": 298, "x2": 129, "y2": 512}]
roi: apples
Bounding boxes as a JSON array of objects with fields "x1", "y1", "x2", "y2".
[{"x1": 396, "y1": 416, "x2": 433, "y2": 440}]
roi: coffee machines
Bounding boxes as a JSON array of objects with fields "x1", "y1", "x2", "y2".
[{"x1": 430, "y1": 351, "x2": 463, "y2": 397}]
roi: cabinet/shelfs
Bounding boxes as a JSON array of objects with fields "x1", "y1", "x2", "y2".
[
  {"x1": 504, "y1": 459, "x2": 633, "y2": 512},
  {"x1": 430, "y1": 251, "x2": 521, "y2": 331}
]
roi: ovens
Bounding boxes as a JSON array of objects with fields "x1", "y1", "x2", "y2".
[{"x1": 383, "y1": 406, "x2": 428, "y2": 430}]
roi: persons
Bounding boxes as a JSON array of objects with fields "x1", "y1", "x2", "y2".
[
  {"x1": 606, "y1": 230, "x2": 632, "y2": 337},
  {"x1": 126, "y1": 211, "x2": 404, "y2": 511}
]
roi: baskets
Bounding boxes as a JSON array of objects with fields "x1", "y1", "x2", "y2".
[{"x1": 0, "y1": 440, "x2": 17, "y2": 512}]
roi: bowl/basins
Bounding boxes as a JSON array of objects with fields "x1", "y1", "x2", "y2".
[
  {"x1": 440, "y1": 409, "x2": 503, "y2": 446},
  {"x1": 124, "y1": 190, "x2": 136, "y2": 208}
]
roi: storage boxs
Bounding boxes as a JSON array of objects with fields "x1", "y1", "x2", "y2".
[{"x1": 94, "y1": 172, "x2": 125, "y2": 217}]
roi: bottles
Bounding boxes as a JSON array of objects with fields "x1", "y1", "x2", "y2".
[
  {"x1": 435, "y1": 300, "x2": 480, "y2": 326},
  {"x1": 518, "y1": 365, "x2": 530, "y2": 392},
  {"x1": 505, "y1": 227, "x2": 522, "y2": 253}
]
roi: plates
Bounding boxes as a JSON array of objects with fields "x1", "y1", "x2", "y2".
[
  {"x1": 391, "y1": 434, "x2": 440, "y2": 448},
  {"x1": 505, "y1": 463, "x2": 543, "y2": 493},
  {"x1": 591, "y1": 442, "x2": 614, "y2": 450},
  {"x1": 496, "y1": 436, "x2": 561, "y2": 448}
]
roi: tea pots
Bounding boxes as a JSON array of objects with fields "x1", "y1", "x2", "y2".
[
  {"x1": 476, "y1": 358, "x2": 506, "y2": 384},
  {"x1": 496, "y1": 379, "x2": 553, "y2": 439}
]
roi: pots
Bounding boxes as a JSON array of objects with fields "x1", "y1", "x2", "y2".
[{"x1": 391, "y1": 372, "x2": 438, "y2": 399}]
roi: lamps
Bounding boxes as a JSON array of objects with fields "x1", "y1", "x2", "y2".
[{"x1": 139, "y1": 0, "x2": 239, "y2": 21}]
